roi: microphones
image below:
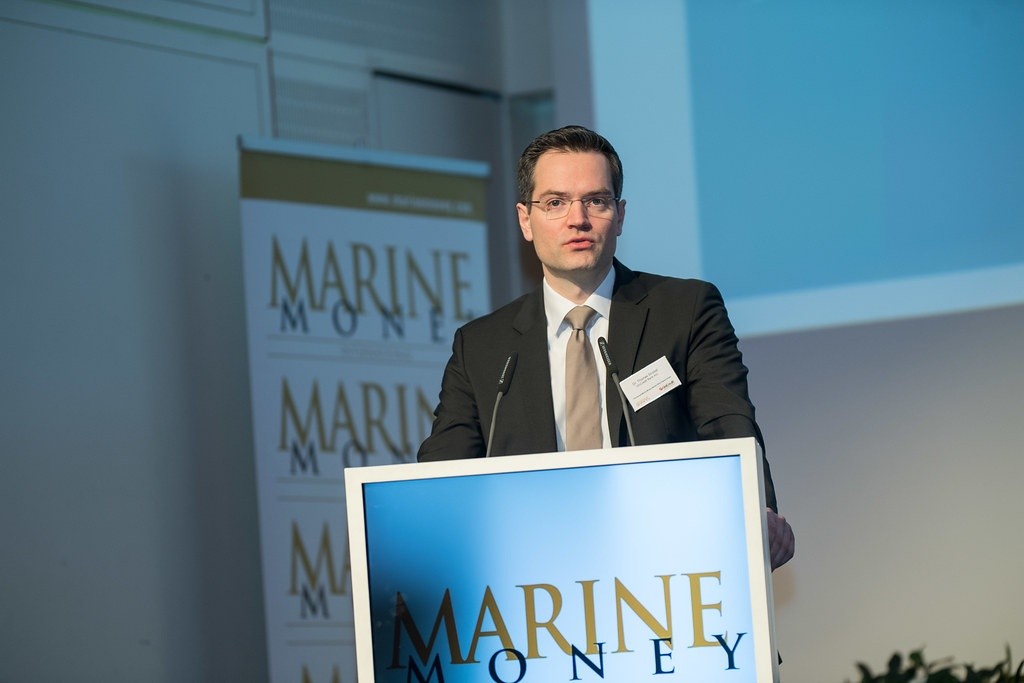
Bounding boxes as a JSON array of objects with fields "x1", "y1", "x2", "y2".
[
  {"x1": 486, "y1": 349, "x2": 520, "y2": 458},
  {"x1": 597, "y1": 337, "x2": 635, "y2": 447}
]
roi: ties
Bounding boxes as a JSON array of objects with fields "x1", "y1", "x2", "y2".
[{"x1": 564, "y1": 306, "x2": 602, "y2": 450}]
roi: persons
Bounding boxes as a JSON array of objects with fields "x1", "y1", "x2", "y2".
[{"x1": 416, "y1": 126, "x2": 794, "y2": 571}]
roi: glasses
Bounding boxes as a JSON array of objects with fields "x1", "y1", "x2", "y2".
[{"x1": 523, "y1": 197, "x2": 619, "y2": 220}]
